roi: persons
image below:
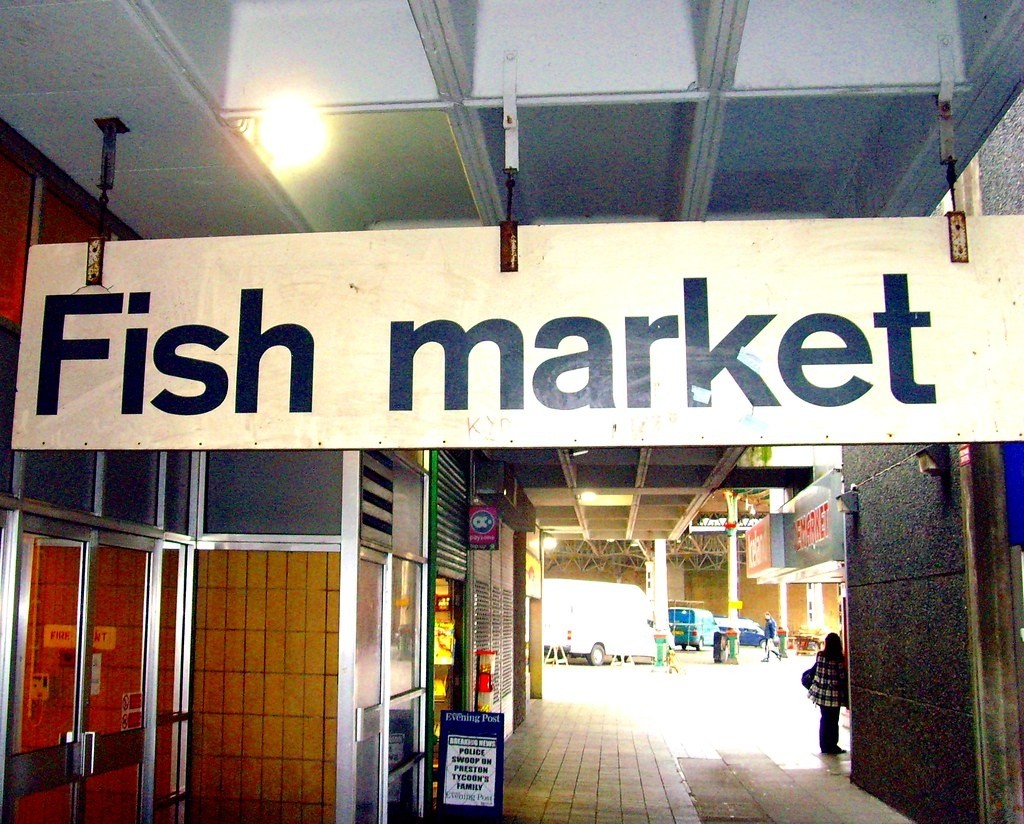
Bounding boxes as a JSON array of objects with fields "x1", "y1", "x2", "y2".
[
  {"x1": 761, "y1": 612, "x2": 782, "y2": 663},
  {"x1": 807, "y1": 633, "x2": 849, "y2": 754}
]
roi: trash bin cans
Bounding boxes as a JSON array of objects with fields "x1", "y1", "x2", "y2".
[{"x1": 713, "y1": 631, "x2": 728, "y2": 664}]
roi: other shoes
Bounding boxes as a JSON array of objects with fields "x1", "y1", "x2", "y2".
[
  {"x1": 761, "y1": 659, "x2": 769, "y2": 662},
  {"x1": 778, "y1": 654, "x2": 781, "y2": 660},
  {"x1": 822, "y1": 746, "x2": 846, "y2": 754}
]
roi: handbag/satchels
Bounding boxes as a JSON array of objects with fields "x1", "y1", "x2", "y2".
[{"x1": 801, "y1": 663, "x2": 818, "y2": 689}]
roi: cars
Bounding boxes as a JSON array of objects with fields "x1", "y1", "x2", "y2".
[{"x1": 716, "y1": 617, "x2": 768, "y2": 649}]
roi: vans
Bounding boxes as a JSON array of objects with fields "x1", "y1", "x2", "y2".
[
  {"x1": 526, "y1": 580, "x2": 675, "y2": 665},
  {"x1": 670, "y1": 604, "x2": 722, "y2": 649}
]
